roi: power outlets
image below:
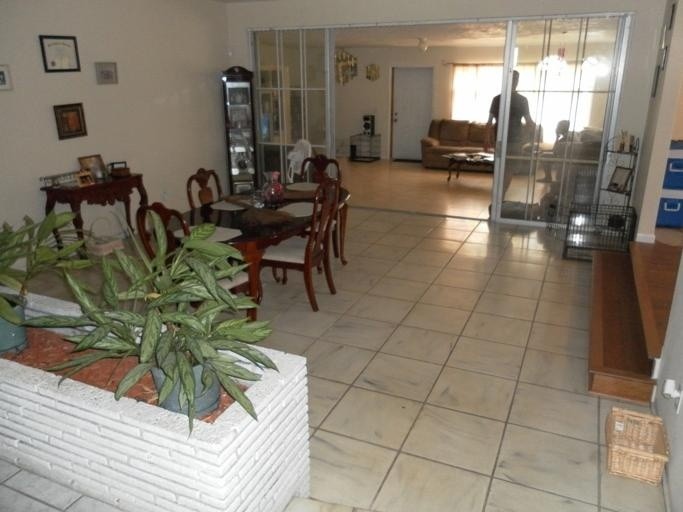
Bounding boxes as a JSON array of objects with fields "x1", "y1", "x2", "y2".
[{"x1": 672, "y1": 382, "x2": 683, "y2": 414}]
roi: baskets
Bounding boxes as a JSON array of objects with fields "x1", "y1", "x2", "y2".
[
  {"x1": 606, "y1": 405, "x2": 671, "y2": 487},
  {"x1": 85, "y1": 216, "x2": 125, "y2": 257}
]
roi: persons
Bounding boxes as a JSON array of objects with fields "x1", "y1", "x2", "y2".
[
  {"x1": 484, "y1": 70, "x2": 536, "y2": 202},
  {"x1": 536, "y1": 119, "x2": 570, "y2": 183}
]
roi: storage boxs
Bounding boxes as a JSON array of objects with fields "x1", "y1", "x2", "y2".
[
  {"x1": 663, "y1": 139, "x2": 683, "y2": 190},
  {"x1": 656, "y1": 187, "x2": 683, "y2": 227}
]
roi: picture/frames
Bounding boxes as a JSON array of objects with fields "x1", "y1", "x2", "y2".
[
  {"x1": 607, "y1": 167, "x2": 633, "y2": 193},
  {"x1": 0, "y1": 34, "x2": 118, "y2": 189}
]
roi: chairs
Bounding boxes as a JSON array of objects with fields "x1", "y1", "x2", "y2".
[
  {"x1": 135, "y1": 202, "x2": 261, "y2": 321},
  {"x1": 187, "y1": 167, "x2": 225, "y2": 209},
  {"x1": 261, "y1": 179, "x2": 341, "y2": 312},
  {"x1": 300, "y1": 154, "x2": 340, "y2": 183}
]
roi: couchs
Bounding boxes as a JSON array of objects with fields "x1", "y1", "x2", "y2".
[
  {"x1": 555, "y1": 125, "x2": 605, "y2": 179},
  {"x1": 420, "y1": 118, "x2": 543, "y2": 174}
]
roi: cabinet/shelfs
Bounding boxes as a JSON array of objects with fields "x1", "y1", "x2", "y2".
[
  {"x1": 592, "y1": 142, "x2": 637, "y2": 243},
  {"x1": 350, "y1": 133, "x2": 381, "y2": 162},
  {"x1": 221, "y1": 65, "x2": 258, "y2": 195},
  {"x1": 260, "y1": 62, "x2": 291, "y2": 141}
]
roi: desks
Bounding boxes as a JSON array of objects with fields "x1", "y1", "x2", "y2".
[{"x1": 40, "y1": 172, "x2": 148, "y2": 265}]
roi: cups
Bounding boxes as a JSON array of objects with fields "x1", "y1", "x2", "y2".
[{"x1": 252, "y1": 190, "x2": 265, "y2": 209}]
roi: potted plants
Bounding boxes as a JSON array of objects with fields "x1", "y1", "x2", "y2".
[
  {"x1": 0, "y1": 212, "x2": 76, "y2": 352},
  {"x1": 18, "y1": 206, "x2": 279, "y2": 438}
]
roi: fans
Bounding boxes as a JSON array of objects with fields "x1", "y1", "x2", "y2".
[{"x1": 285, "y1": 139, "x2": 311, "y2": 183}]
